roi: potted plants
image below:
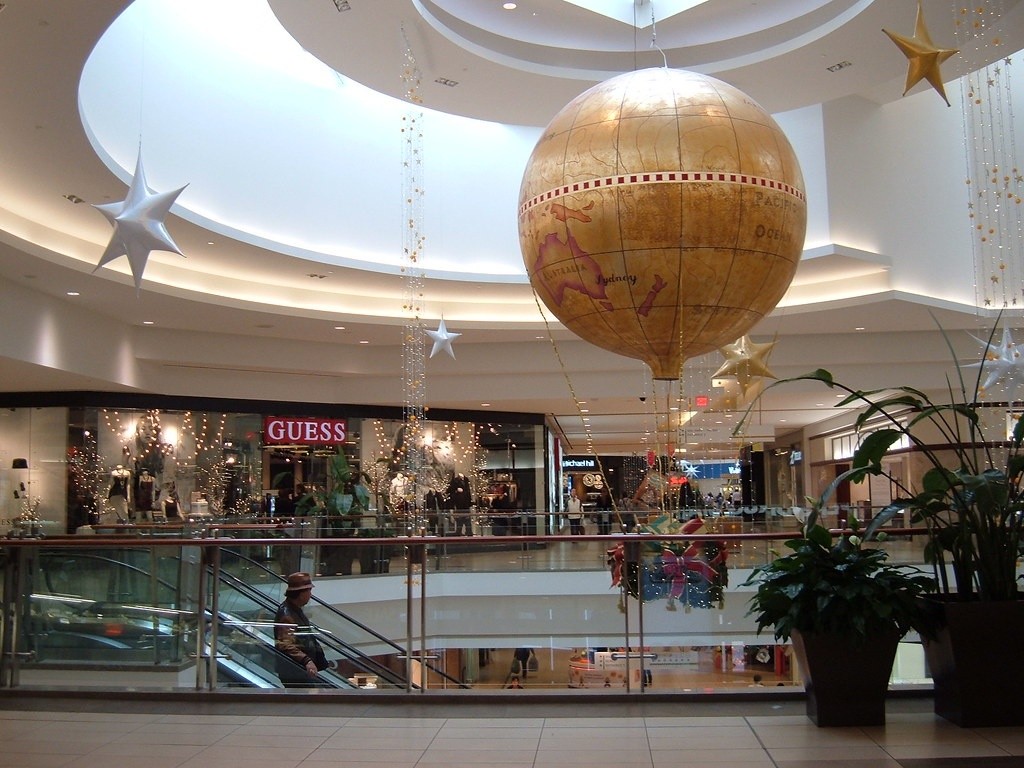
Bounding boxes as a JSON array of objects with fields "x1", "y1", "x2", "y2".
[
  {"x1": 732, "y1": 306, "x2": 1024, "y2": 728},
  {"x1": 294, "y1": 443, "x2": 398, "y2": 576}
]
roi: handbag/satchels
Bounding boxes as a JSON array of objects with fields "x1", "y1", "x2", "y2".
[
  {"x1": 529, "y1": 655, "x2": 539, "y2": 671},
  {"x1": 510, "y1": 656, "x2": 521, "y2": 674}
]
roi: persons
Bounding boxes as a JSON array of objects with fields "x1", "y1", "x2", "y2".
[
  {"x1": 749, "y1": 674, "x2": 763, "y2": 688},
  {"x1": 643, "y1": 648, "x2": 652, "y2": 688},
  {"x1": 154, "y1": 480, "x2": 168, "y2": 519},
  {"x1": 564, "y1": 488, "x2": 585, "y2": 543},
  {"x1": 426, "y1": 487, "x2": 445, "y2": 537},
  {"x1": 507, "y1": 675, "x2": 524, "y2": 689},
  {"x1": 134, "y1": 470, "x2": 153, "y2": 522},
  {"x1": 449, "y1": 472, "x2": 474, "y2": 536},
  {"x1": 273, "y1": 482, "x2": 313, "y2": 517},
  {"x1": 274, "y1": 571, "x2": 330, "y2": 689},
  {"x1": 389, "y1": 473, "x2": 409, "y2": 504},
  {"x1": 663, "y1": 489, "x2": 742, "y2": 512},
  {"x1": 514, "y1": 648, "x2": 535, "y2": 679},
  {"x1": 105, "y1": 465, "x2": 132, "y2": 525},
  {"x1": 135, "y1": 414, "x2": 160, "y2": 451}
]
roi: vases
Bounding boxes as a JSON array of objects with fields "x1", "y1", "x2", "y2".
[{"x1": 789, "y1": 621, "x2": 900, "y2": 727}]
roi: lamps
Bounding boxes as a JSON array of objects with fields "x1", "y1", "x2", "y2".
[{"x1": 12, "y1": 459, "x2": 29, "y2": 469}]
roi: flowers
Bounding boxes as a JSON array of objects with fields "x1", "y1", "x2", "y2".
[{"x1": 734, "y1": 495, "x2": 951, "y2": 653}]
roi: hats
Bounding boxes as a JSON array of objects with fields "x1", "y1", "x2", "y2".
[{"x1": 285, "y1": 572, "x2": 315, "y2": 592}]
